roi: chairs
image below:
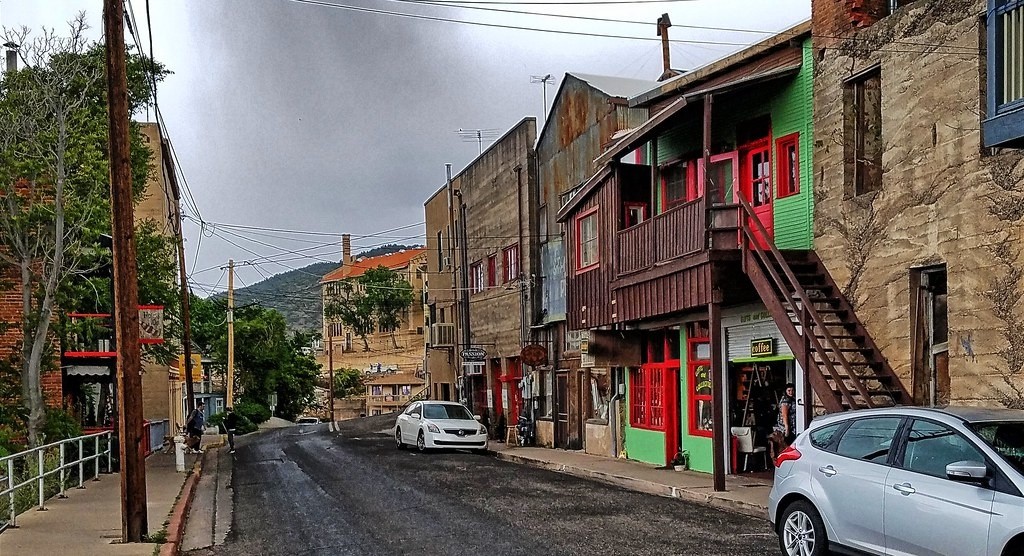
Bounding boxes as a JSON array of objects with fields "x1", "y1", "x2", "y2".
[{"x1": 731, "y1": 427, "x2": 768, "y2": 473}]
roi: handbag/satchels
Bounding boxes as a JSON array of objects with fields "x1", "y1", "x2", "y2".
[{"x1": 187, "y1": 409, "x2": 199, "y2": 433}]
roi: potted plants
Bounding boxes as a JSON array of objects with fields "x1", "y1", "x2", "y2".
[{"x1": 670, "y1": 450, "x2": 688, "y2": 472}]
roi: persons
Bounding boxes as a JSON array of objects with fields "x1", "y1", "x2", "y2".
[
  {"x1": 223, "y1": 407, "x2": 237, "y2": 453},
  {"x1": 777, "y1": 383, "x2": 796, "y2": 446},
  {"x1": 185, "y1": 402, "x2": 205, "y2": 454}
]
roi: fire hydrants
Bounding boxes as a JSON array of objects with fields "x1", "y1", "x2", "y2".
[{"x1": 173, "y1": 436, "x2": 189, "y2": 473}]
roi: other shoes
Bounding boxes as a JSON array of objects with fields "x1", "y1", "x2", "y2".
[
  {"x1": 229, "y1": 450, "x2": 235, "y2": 453},
  {"x1": 198, "y1": 449, "x2": 204, "y2": 454},
  {"x1": 191, "y1": 448, "x2": 198, "y2": 453}
]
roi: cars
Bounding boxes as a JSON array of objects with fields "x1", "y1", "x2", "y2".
[
  {"x1": 768, "y1": 405, "x2": 1023, "y2": 554},
  {"x1": 394, "y1": 400, "x2": 491, "y2": 453},
  {"x1": 297, "y1": 417, "x2": 322, "y2": 427}
]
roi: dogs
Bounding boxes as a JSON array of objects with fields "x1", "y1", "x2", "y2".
[{"x1": 163, "y1": 435, "x2": 200, "y2": 454}]
